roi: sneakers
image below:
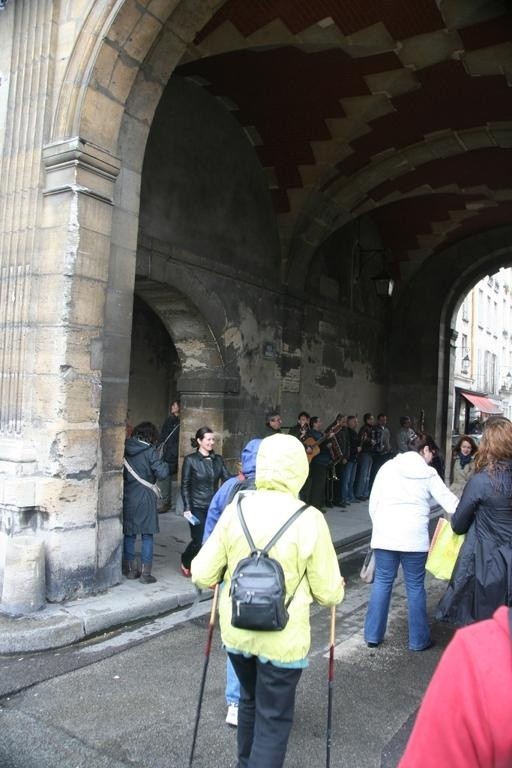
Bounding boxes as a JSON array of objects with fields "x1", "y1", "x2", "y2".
[
  {"x1": 421, "y1": 640, "x2": 434, "y2": 652},
  {"x1": 224, "y1": 702, "x2": 239, "y2": 727},
  {"x1": 368, "y1": 641, "x2": 376, "y2": 647},
  {"x1": 314, "y1": 494, "x2": 369, "y2": 515}
]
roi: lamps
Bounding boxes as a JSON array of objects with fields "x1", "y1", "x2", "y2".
[{"x1": 373, "y1": 276, "x2": 395, "y2": 296}]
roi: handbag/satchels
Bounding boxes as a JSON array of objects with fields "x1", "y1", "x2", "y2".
[
  {"x1": 358, "y1": 547, "x2": 398, "y2": 585},
  {"x1": 423, "y1": 516, "x2": 466, "y2": 582},
  {"x1": 154, "y1": 479, "x2": 162, "y2": 501}
]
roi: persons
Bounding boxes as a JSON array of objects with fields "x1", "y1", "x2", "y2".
[
  {"x1": 362, "y1": 430, "x2": 460, "y2": 653},
  {"x1": 121, "y1": 420, "x2": 171, "y2": 583},
  {"x1": 189, "y1": 433, "x2": 345, "y2": 768},
  {"x1": 263, "y1": 411, "x2": 445, "y2": 512},
  {"x1": 179, "y1": 425, "x2": 234, "y2": 576},
  {"x1": 396, "y1": 601, "x2": 512, "y2": 767},
  {"x1": 155, "y1": 400, "x2": 181, "y2": 514},
  {"x1": 442, "y1": 436, "x2": 479, "y2": 522},
  {"x1": 200, "y1": 438, "x2": 260, "y2": 725},
  {"x1": 432, "y1": 416, "x2": 511, "y2": 628}
]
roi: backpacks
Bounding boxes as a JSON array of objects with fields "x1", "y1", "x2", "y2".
[{"x1": 229, "y1": 493, "x2": 312, "y2": 633}]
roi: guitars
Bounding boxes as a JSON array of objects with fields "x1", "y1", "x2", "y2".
[{"x1": 302, "y1": 424, "x2": 341, "y2": 464}]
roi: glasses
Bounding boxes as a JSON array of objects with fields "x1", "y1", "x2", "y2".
[{"x1": 430, "y1": 449, "x2": 438, "y2": 457}]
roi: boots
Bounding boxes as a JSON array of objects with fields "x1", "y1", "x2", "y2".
[
  {"x1": 124, "y1": 558, "x2": 142, "y2": 580},
  {"x1": 139, "y1": 559, "x2": 157, "y2": 585}
]
